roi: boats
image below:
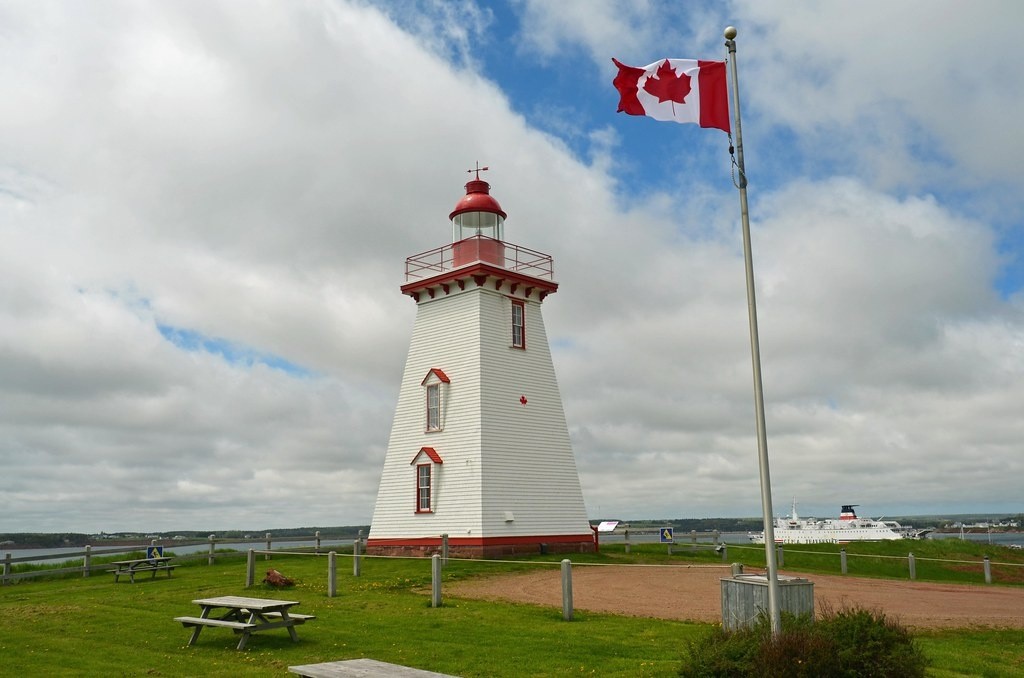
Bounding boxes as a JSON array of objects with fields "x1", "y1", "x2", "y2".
[{"x1": 747, "y1": 504, "x2": 933, "y2": 545}]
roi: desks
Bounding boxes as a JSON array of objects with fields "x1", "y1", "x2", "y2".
[
  {"x1": 111, "y1": 556, "x2": 175, "y2": 583},
  {"x1": 287, "y1": 658, "x2": 462, "y2": 678},
  {"x1": 187, "y1": 596, "x2": 299, "y2": 650}
]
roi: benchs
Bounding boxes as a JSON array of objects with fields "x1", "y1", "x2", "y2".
[
  {"x1": 127, "y1": 564, "x2": 182, "y2": 575},
  {"x1": 173, "y1": 616, "x2": 257, "y2": 634},
  {"x1": 107, "y1": 565, "x2": 154, "y2": 573},
  {"x1": 239, "y1": 608, "x2": 316, "y2": 623}
]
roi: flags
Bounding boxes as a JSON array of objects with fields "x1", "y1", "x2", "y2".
[{"x1": 612, "y1": 57, "x2": 731, "y2": 133}]
